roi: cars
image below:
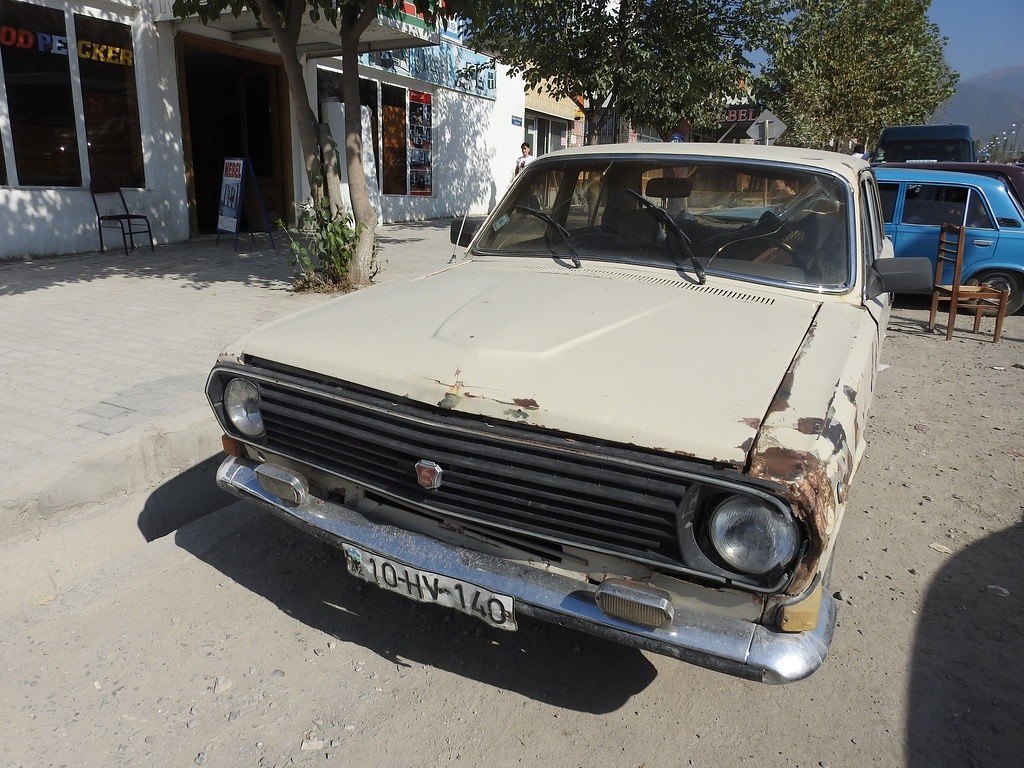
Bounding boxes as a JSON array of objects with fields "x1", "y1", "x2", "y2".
[
  {"x1": 878, "y1": 160, "x2": 1024, "y2": 211},
  {"x1": 204, "y1": 142, "x2": 935, "y2": 686},
  {"x1": 696, "y1": 167, "x2": 1024, "y2": 318}
]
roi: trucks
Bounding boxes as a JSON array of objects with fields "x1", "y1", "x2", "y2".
[{"x1": 860, "y1": 124, "x2": 976, "y2": 162}]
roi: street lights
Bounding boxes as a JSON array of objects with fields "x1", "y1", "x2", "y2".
[{"x1": 1012, "y1": 120, "x2": 1024, "y2": 159}]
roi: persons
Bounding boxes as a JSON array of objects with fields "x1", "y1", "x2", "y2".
[
  {"x1": 662, "y1": 134, "x2": 698, "y2": 209},
  {"x1": 850, "y1": 146, "x2": 870, "y2": 160},
  {"x1": 515, "y1": 142, "x2": 536, "y2": 175}
]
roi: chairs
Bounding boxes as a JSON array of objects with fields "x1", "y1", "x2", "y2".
[
  {"x1": 733, "y1": 210, "x2": 837, "y2": 283},
  {"x1": 929, "y1": 222, "x2": 1009, "y2": 344},
  {"x1": 89, "y1": 177, "x2": 156, "y2": 256},
  {"x1": 601, "y1": 206, "x2": 667, "y2": 250}
]
